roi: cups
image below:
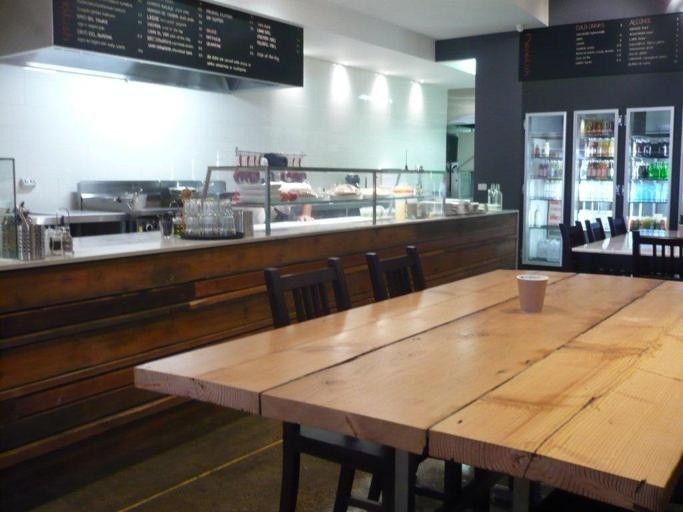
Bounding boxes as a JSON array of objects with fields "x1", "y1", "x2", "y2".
[
  {"x1": 183, "y1": 197, "x2": 236, "y2": 236},
  {"x1": 516, "y1": 275, "x2": 548, "y2": 312}
]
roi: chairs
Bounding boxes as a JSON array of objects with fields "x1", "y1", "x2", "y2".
[
  {"x1": 559, "y1": 216, "x2": 683, "y2": 282},
  {"x1": 367, "y1": 246, "x2": 487, "y2": 500},
  {"x1": 264, "y1": 258, "x2": 461, "y2": 512}
]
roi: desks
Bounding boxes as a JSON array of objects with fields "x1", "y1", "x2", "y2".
[{"x1": 133, "y1": 269, "x2": 683, "y2": 512}]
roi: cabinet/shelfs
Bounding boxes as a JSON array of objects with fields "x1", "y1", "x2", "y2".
[{"x1": 198, "y1": 164, "x2": 451, "y2": 235}]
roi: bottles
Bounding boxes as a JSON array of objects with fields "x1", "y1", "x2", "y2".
[
  {"x1": 531, "y1": 117, "x2": 669, "y2": 179},
  {"x1": 45, "y1": 227, "x2": 74, "y2": 257},
  {"x1": 488, "y1": 183, "x2": 503, "y2": 212},
  {"x1": 159, "y1": 213, "x2": 182, "y2": 239}
]
